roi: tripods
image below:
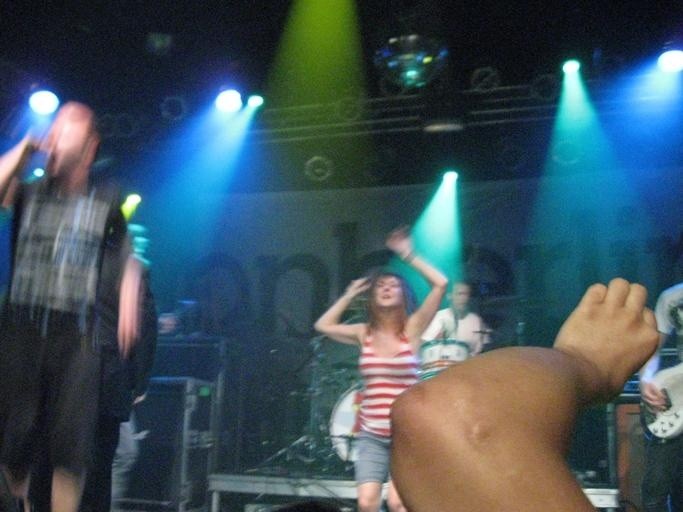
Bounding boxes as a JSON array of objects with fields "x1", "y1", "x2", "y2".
[{"x1": 246, "y1": 365, "x2": 357, "y2": 478}]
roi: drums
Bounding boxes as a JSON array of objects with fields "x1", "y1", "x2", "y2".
[
  {"x1": 328, "y1": 381, "x2": 359, "y2": 462},
  {"x1": 421, "y1": 339, "x2": 470, "y2": 381}
]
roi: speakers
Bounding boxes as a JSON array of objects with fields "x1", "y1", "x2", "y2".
[
  {"x1": 121, "y1": 377, "x2": 214, "y2": 509},
  {"x1": 149, "y1": 337, "x2": 245, "y2": 475}
]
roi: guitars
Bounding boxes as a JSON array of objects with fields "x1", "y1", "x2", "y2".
[{"x1": 638, "y1": 362, "x2": 683, "y2": 444}]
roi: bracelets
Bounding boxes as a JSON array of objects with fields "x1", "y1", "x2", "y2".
[{"x1": 402, "y1": 252, "x2": 416, "y2": 264}]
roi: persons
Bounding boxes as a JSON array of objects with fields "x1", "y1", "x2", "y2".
[
  {"x1": 389, "y1": 277, "x2": 659, "y2": 512},
  {"x1": 420, "y1": 283, "x2": 492, "y2": 357},
  {"x1": 639, "y1": 282, "x2": 683, "y2": 512},
  {"x1": 313, "y1": 228, "x2": 448, "y2": 512},
  {"x1": 0, "y1": 101, "x2": 145, "y2": 512},
  {"x1": 30, "y1": 263, "x2": 155, "y2": 512}
]
